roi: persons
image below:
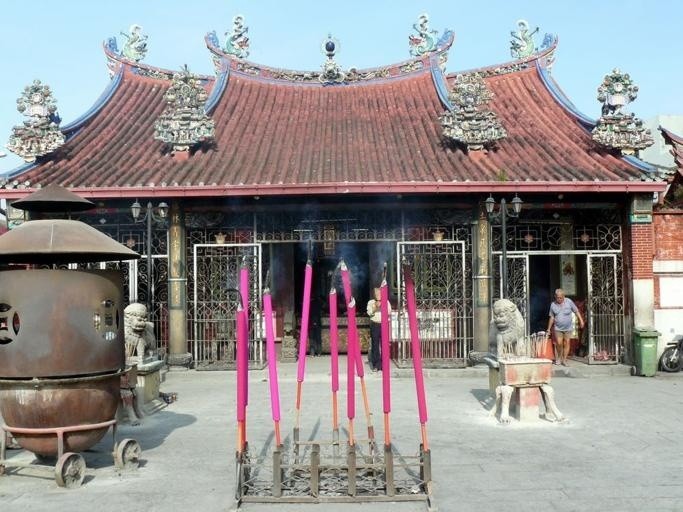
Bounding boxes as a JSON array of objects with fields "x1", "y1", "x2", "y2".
[
  {"x1": 546, "y1": 288, "x2": 584, "y2": 366},
  {"x1": 294, "y1": 291, "x2": 327, "y2": 358},
  {"x1": 365, "y1": 287, "x2": 392, "y2": 371}
]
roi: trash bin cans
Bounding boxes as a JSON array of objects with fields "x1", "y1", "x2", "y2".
[{"x1": 631, "y1": 327, "x2": 662, "y2": 377}]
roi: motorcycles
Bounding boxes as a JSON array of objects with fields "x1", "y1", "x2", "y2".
[{"x1": 660, "y1": 336, "x2": 682, "y2": 371}]
